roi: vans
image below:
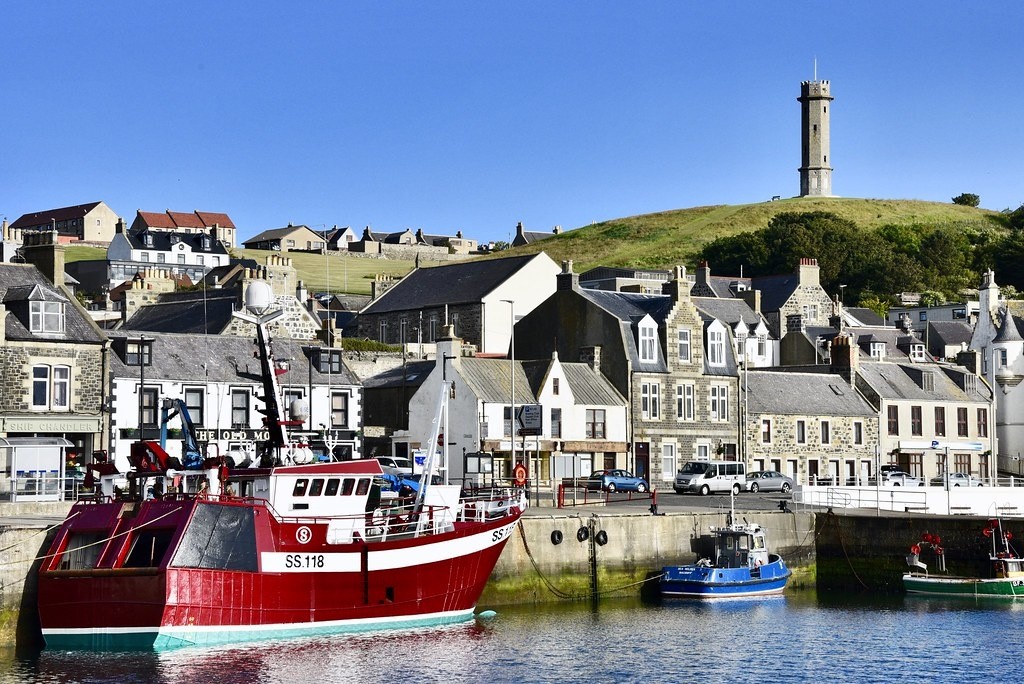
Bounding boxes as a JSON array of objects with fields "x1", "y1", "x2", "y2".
[
  {"x1": 673, "y1": 461, "x2": 746, "y2": 495},
  {"x1": 372, "y1": 455, "x2": 413, "y2": 477}
]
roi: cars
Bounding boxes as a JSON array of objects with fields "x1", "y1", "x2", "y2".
[
  {"x1": 930, "y1": 472, "x2": 989, "y2": 488},
  {"x1": 588, "y1": 469, "x2": 648, "y2": 493},
  {"x1": 399, "y1": 474, "x2": 468, "y2": 510},
  {"x1": 869, "y1": 471, "x2": 925, "y2": 487},
  {"x1": 745, "y1": 471, "x2": 793, "y2": 493}
]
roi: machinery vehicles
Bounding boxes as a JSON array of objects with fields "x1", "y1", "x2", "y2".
[{"x1": 160, "y1": 398, "x2": 203, "y2": 471}]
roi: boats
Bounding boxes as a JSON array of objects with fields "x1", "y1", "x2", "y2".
[
  {"x1": 902, "y1": 518, "x2": 1024, "y2": 597},
  {"x1": 660, "y1": 481, "x2": 792, "y2": 598},
  {"x1": 40, "y1": 280, "x2": 527, "y2": 653}
]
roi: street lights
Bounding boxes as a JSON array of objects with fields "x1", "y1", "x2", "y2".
[{"x1": 500, "y1": 300, "x2": 516, "y2": 488}]
